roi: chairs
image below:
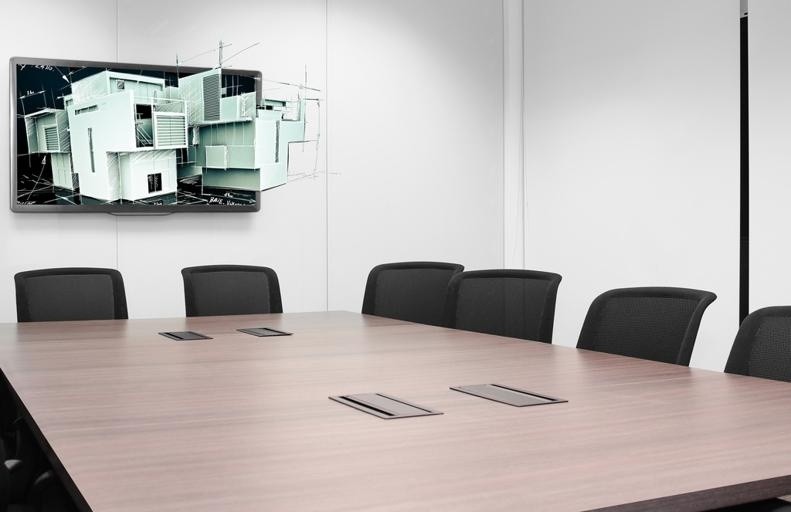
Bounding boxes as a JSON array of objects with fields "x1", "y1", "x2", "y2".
[
  {"x1": 722, "y1": 305, "x2": 789, "y2": 383},
  {"x1": 575, "y1": 286, "x2": 717, "y2": 367},
  {"x1": 181, "y1": 264, "x2": 284, "y2": 316},
  {"x1": 361, "y1": 261, "x2": 465, "y2": 329},
  {"x1": 1, "y1": 441, "x2": 78, "y2": 511},
  {"x1": 14, "y1": 267, "x2": 129, "y2": 325},
  {"x1": 442, "y1": 269, "x2": 562, "y2": 347}
]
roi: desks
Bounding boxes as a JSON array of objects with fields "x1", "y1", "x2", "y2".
[{"x1": 1, "y1": 310, "x2": 789, "y2": 510}]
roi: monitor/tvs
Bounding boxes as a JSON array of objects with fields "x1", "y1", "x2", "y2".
[{"x1": 7, "y1": 56, "x2": 263, "y2": 215}]
